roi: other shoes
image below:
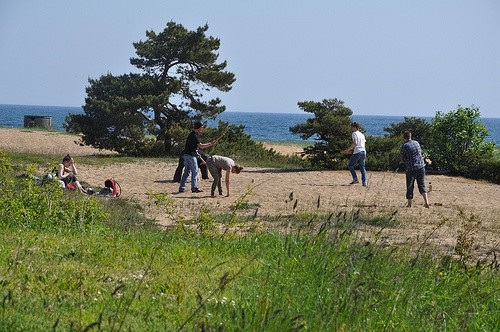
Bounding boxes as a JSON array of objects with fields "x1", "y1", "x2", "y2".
[
  {"x1": 192, "y1": 188, "x2": 203, "y2": 193},
  {"x1": 349, "y1": 180, "x2": 360, "y2": 184}
]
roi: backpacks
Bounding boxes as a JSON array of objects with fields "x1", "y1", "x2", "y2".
[{"x1": 104, "y1": 177, "x2": 121, "y2": 197}]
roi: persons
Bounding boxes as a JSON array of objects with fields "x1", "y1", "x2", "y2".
[
  {"x1": 53, "y1": 155, "x2": 87, "y2": 193},
  {"x1": 207, "y1": 155, "x2": 243, "y2": 197},
  {"x1": 173, "y1": 122, "x2": 217, "y2": 192},
  {"x1": 343, "y1": 122, "x2": 369, "y2": 186},
  {"x1": 398, "y1": 132, "x2": 430, "y2": 208}
]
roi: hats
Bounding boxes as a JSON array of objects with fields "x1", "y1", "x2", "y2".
[{"x1": 195, "y1": 121, "x2": 203, "y2": 129}]
